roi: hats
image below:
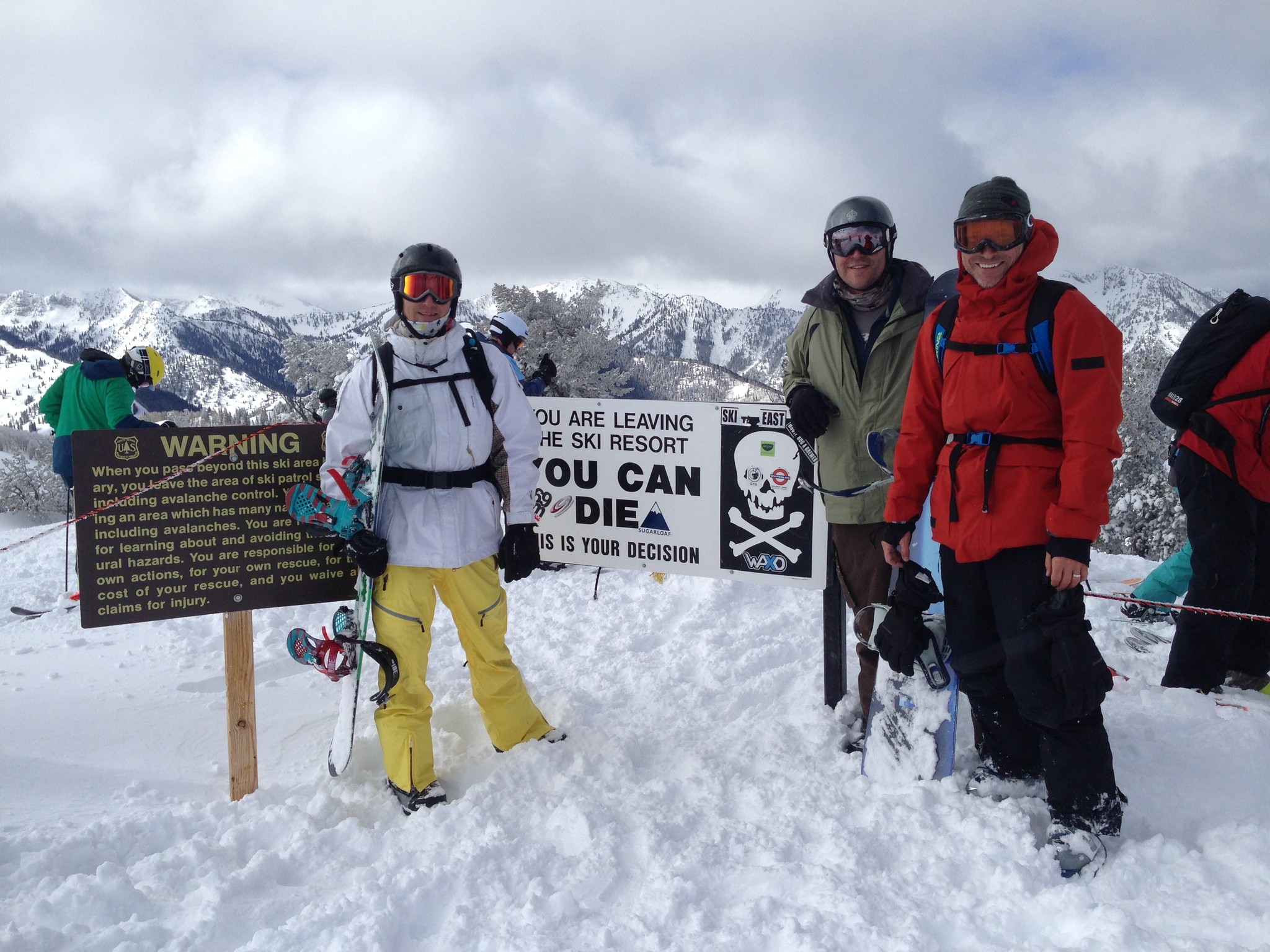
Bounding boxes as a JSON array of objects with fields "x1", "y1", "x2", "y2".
[{"x1": 957, "y1": 176, "x2": 1030, "y2": 218}]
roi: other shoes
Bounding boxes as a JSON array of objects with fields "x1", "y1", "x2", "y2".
[
  {"x1": 1223, "y1": 670, "x2": 1270, "y2": 696},
  {"x1": 1191, "y1": 685, "x2": 1224, "y2": 695}
]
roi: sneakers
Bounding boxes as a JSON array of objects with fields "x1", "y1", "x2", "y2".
[
  {"x1": 966, "y1": 775, "x2": 1047, "y2": 802},
  {"x1": 1046, "y1": 824, "x2": 1108, "y2": 879},
  {"x1": 491, "y1": 729, "x2": 568, "y2": 753},
  {"x1": 388, "y1": 780, "x2": 448, "y2": 816},
  {"x1": 1120, "y1": 596, "x2": 1181, "y2": 624}
]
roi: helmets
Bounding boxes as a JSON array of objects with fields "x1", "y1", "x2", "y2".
[
  {"x1": 390, "y1": 242, "x2": 463, "y2": 315},
  {"x1": 824, "y1": 195, "x2": 898, "y2": 262},
  {"x1": 125, "y1": 345, "x2": 165, "y2": 391},
  {"x1": 489, "y1": 313, "x2": 529, "y2": 347}
]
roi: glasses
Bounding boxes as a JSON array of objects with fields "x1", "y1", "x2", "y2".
[
  {"x1": 826, "y1": 226, "x2": 890, "y2": 258},
  {"x1": 953, "y1": 215, "x2": 1027, "y2": 253},
  {"x1": 318, "y1": 388, "x2": 338, "y2": 402},
  {"x1": 514, "y1": 337, "x2": 525, "y2": 351},
  {"x1": 139, "y1": 380, "x2": 151, "y2": 389},
  {"x1": 317, "y1": 398, "x2": 323, "y2": 403},
  {"x1": 399, "y1": 271, "x2": 458, "y2": 305}
]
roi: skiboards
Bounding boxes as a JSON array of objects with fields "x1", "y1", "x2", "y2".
[
  {"x1": 1106, "y1": 665, "x2": 1250, "y2": 715},
  {"x1": 10, "y1": 605, "x2": 78, "y2": 625},
  {"x1": 1124, "y1": 626, "x2": 1180, "y2": 653},
  {"x1": 1084, "y1": 615, "x2": 1171, "y2": 627}
]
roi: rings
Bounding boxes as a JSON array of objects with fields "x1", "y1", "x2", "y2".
[{"x1": 1073, "y1": 574, "x2": 1081, "y2": 577}]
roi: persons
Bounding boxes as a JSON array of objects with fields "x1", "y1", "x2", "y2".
[
  {"x1": 38, "y1": 346, "x2": 164, "y2": 573},
  {"x1": 1119, "y1": 289, "x2": 1270, "y2": 697},
  {"x1": 320, "y1": 242, "x2": 566, "y2": 818},
  {"x1": 783, "y1": 195, "x2": 948, "y2": 683},
  {"x1": 878, "y1": 177, "x2": 1128, "y2": 875}
]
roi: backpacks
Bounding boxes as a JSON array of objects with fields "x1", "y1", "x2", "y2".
[{"x1": 1149, "y1": 288, "x2": 1270, "y2": 495}]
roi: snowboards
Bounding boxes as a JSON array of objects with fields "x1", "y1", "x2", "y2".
[
  {"x1": 1118, "y1": 578, "x2": 1147, "y2": 585},
  {"x1": 328, "y1": 327, "x2": 390, "y2": 776},
  {"x1": 1109, "y1": 591, "x2": 1133, "y2": 596},
  {"x1": 861, "y1": 481, "x2": 960, "y2": 783}
]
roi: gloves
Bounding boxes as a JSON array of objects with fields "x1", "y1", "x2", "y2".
[
  {"x1": 498, "y1": 523, "x2": 540, "y2": 583},
  {"x1": 790, "y1": 385, "x2": 841, "y2": 441},
  {"x1": 344, "y1": 529, "x2": 389, "y2": 578},
  {"x1": 161, "y1": 421, "x2": 178, "y2": 428},
  {"x1": 874, "y1": 559, "x2": 945, "y2": 677},
  {"x1": 532, "y1": 353, "x2": 558, "y2": 386}
]
